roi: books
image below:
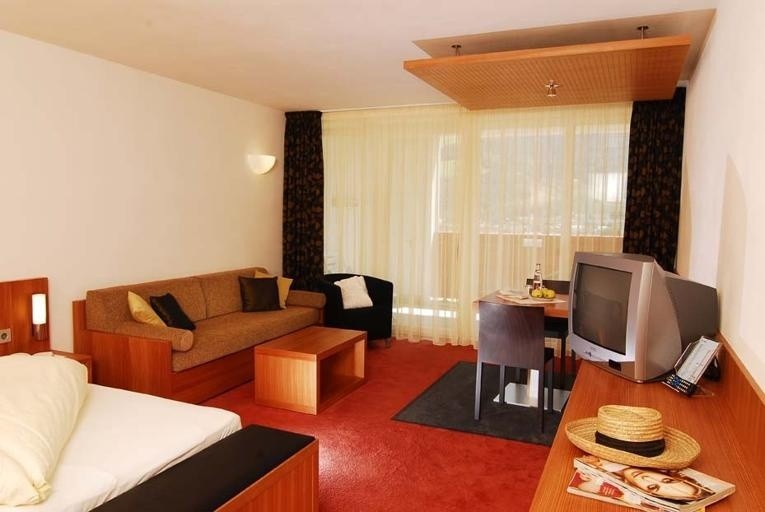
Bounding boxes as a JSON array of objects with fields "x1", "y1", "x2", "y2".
[{"x1": 564, "y1": 453, "x2": 737, "y2": 512}]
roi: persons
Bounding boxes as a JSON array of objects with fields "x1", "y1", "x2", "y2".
[{"x1": 580, "y1": 456, "x2": 704, "y2": 505}]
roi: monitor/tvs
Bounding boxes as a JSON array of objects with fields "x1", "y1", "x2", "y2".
[{"x1": 568, "y1": 252, "x2": 719, "y2": 385}]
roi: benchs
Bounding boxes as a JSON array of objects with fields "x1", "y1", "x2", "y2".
[{"x1": 89, "y1": 422, "x2": 319, "y2": 511}]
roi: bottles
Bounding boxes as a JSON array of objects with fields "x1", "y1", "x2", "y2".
[{"x1": 533, "y1": 264, "x2": 542, "y2": 289}]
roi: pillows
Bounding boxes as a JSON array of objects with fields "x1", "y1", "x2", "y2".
[
  {"x1": 124, "y1": 289, "x2": 197, "y2": 331},
  {"x1": 236, "y1": 270, "x2": 295, "y2": 313},
  {"x1": 333, "y1": 276, "x2": 373, "y2": 310}
]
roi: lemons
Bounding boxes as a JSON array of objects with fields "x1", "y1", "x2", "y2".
[{"x1": 532, "y1": 288, "x2": 555, "y2": 298}]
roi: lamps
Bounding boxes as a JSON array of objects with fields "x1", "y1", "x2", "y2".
[
  {"x1": 31, "y1": 293, "x2": 47, "y2": 342},
  {"x1": 546, "y1": 80, "x2": 561, "y2": 98},
  {"x1": 248, "y1": 153, "x2": 278, "y2": 176}
]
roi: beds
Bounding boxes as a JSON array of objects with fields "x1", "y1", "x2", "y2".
[{"x1": 0, "y1": 276, "x2": 243, "y2": 509}]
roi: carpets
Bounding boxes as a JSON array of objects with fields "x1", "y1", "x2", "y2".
[{"x1": 389, "y1": 360, "x2": 575, "y2": 449}]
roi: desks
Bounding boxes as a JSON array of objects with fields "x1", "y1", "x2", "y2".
[{"x1": 526, "y1": 359, "x2": 765, "y2": 511}]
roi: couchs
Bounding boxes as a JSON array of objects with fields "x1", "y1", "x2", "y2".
[{"x1": 72, "y1": 265, "x2": 327, "y2": 405}]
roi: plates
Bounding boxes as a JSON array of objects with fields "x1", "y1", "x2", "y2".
[{"x1": 531, "y1": 296, "x2": 555, "y2": 302}]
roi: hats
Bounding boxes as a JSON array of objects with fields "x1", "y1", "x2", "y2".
[{"x1": 564, "y1": 404, "x2": 701, "y2": 469}]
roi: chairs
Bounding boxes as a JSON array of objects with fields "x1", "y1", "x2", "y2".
[
  {"x1": 473, "y1": 277, "x2": 577, "y2": 434},
  {"x1": 319, "y1": 273, "x2": 393, "y2": 348}
]
